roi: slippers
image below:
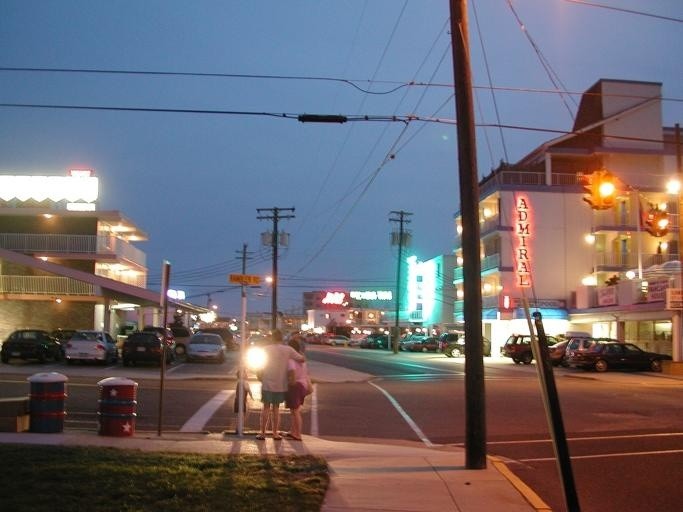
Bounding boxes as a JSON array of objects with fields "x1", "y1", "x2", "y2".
[{"x1": 256, "y1": 432, "x2": 303, "y2": 441}]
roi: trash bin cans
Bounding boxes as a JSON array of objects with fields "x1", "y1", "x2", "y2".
[
  {"x1": 26, "y1": 371, "x2": 68, "y2": 433},
  {"x1": 96, "y1": 376, "x2": 139, "y2": 437}
]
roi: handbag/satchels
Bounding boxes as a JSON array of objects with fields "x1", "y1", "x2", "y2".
[{"x1": 306, "y1": 377, "x2": 313, "y2": 396}]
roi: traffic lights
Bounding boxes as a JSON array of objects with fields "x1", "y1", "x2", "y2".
[
  {"x1": 579, "y1": 172, "x2": 621, "y2": 210},
  {"x1": 643, "y1": 211, "x2": 673, "y2": 238}
]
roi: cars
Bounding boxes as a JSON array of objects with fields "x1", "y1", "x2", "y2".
[
  {"x1": 123, "y1": 325, "x2": 236, "y2": 367},
  {"x1": 287, "y1": 329, "x2": 490, "y2": 359},
  {"x1": 1, "y1": 328, "x2": 117, "y2": 366}
]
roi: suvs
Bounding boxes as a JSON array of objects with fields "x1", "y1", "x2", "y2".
[{"x1": 501, "y1": 334, "x2": 672, "y2": 373}]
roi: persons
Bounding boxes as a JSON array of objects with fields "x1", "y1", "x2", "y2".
[
  {"x1": 287, "y1": 338, "x2": 309, "y2": 440},
  {"x1": 256, "y1": 328, "x2": 305, "y2": 440},
  {"x1": 233, "y1": 370, "x2": 254, "y2": 433}
]
